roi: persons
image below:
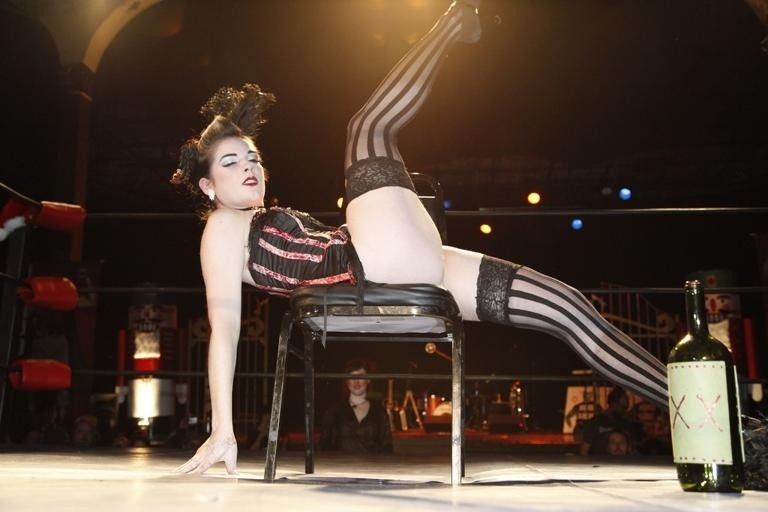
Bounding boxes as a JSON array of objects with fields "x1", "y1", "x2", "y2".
[
  {"x1": 19, "y1": 393, "x2": 149, "y2": 446},
  {"x1": 578, "y1": 385, "x2": 673, "y2": 457},
  {"x1": 315, "y1": 355, "x2": 393, "y2": 453},
  {"x1": 169, "y1": 0, "x2": 762, "y2": 476}
]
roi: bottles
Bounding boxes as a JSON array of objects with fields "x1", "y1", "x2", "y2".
[{"x1": 665, "y1": 279, "x2": 748, "y2": 493}]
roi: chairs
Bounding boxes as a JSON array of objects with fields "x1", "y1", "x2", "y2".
[{"x1": 264, "y1": 173, "x2": 466, "y2": 480}]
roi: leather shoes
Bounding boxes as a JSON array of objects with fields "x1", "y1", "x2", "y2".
[{"x1": 456, "y1": 0, "x2": 502, "y2": 59}]
